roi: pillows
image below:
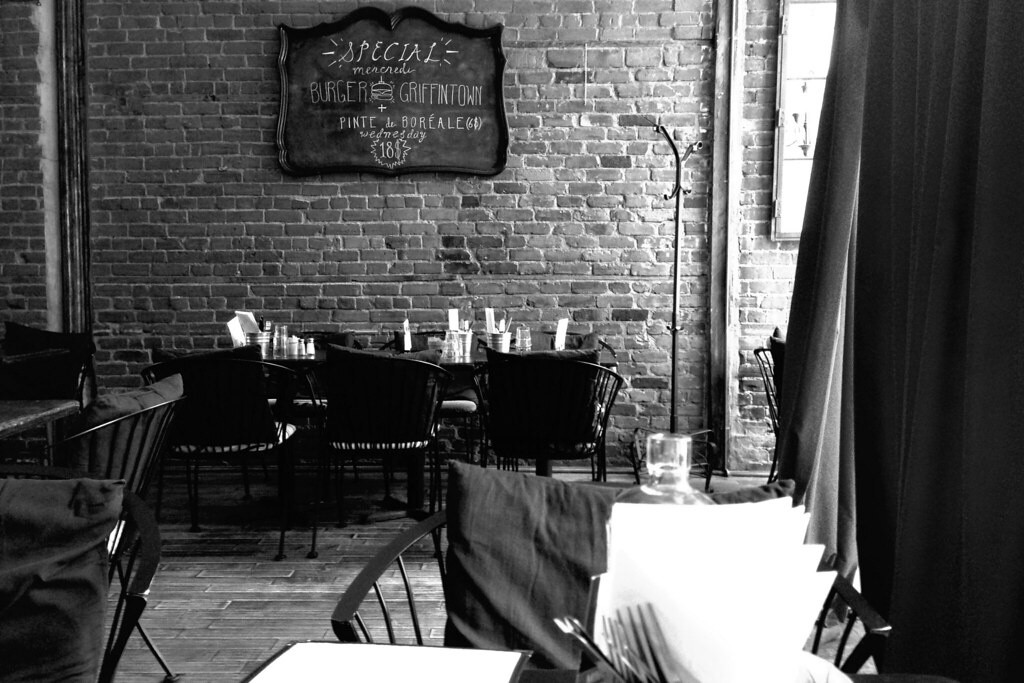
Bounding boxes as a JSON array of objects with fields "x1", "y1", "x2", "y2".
[
  {"x1": 0, "y1": 478, "x2": 127, "y2": 683},
  {"x1": 443, "y1": 457, "x2": 796, "y2": 669}
]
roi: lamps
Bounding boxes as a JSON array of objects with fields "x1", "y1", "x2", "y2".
[{"x1": 780, "y1": 82, "x2": 823, "y2": 158}]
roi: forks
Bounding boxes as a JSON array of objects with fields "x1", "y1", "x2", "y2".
[{"x1": 553, "y1": 600, "x2": 699, "y2": 683}]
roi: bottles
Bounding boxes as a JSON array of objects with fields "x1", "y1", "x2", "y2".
[
  {"x1": 245, "y1": 325, "x2": 315, "y2": 360},
  {"x1": 612, "y1": 434, "x2": 719, "y2": 504}
]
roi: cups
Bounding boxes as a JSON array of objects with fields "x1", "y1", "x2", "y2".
[{"x1": 440, "y1": 326, "x2": 533, "y2": 362}]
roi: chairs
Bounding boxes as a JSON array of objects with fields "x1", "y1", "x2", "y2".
[
  {"x1": 0, "y1": 461, "x2": 184, "y2": 682},
  {"x1": 752, "y1": 347, "x2": 789, "y2": 488},
  {"x1": 331, "y1": 481, "x2": 893, "y2": 674},
  {"x1": 0, "y1": 311, "x2": 626, "y2": 556}
]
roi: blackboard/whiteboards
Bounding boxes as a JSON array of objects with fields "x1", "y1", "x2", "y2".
[{"x1": 276, "y1": 4, "x2": 511, "y2": 177}]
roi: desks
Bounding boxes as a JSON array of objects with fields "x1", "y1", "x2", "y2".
[{"x1": 239, "y1": 635, "x2": 967, "y2": 683}]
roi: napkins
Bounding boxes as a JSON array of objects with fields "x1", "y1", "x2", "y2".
[{"x1": 593, "y1": 494, "x2": 839, "y2": 683}]
